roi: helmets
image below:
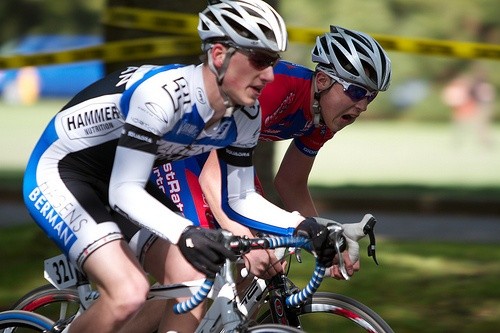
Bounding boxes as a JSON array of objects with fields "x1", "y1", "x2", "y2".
[
  {"x1": 197, "y1": 0, "x2": 287, "y2": 52},
  {"x1": 311, "y1": 24, "x2": 392, "y2": 91}
]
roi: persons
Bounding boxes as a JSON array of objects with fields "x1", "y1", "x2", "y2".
[
  {"x1": 119, "y1": 25, "x2": 392, "y2": 333},
  {"x1": 22, "y1": 0, "x2": 354, "y2": 332}
]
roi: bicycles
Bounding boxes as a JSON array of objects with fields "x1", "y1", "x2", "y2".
[
  {"x1": 3, "y1": 213, "x2": 397, "y2": 331},
  {"x1": 0, "y1": 235, "x2": 326, "y2": 333}
]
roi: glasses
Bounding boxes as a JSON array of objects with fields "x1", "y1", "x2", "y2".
[
  {"x1": 216, "y1": 40, "x2": 281, "y2": 70},
  {"x1": 317, "y1": 66, "x2": 379, "y2": 103}
]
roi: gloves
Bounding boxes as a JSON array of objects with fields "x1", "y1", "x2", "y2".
[
  {"x1": 293, "y1": 216, "x2": 346, "y2": 268},
  {"x1": 177, "y1": 226, "x2": 237, "y2": 277}
]
